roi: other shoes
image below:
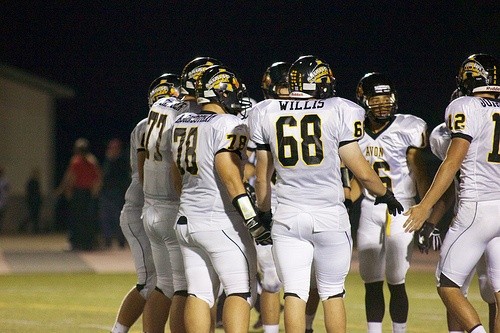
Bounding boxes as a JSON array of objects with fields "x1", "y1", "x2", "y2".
[{"x1": 252, "y1": 305, "x2": 284, "y2": 328}]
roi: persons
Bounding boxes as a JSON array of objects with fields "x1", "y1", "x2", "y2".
[
  {"x1": 344, "y1": 72, "x2": 444, "y2": 333},
  {"x1": 112, "y1": 72, "x2": 180, "y2": 333},
  {"x1": 103, "y1": 140, "x2": 131, "y2": 247},
  {"x1": 17, "y1": 169, "x2": 41, "y2": 235},
  {"x1": 167, "y1": 55, "x2": 467, "y2": 333},
  {"x1": 171, "y1": 65, "x2": 273, "y2": 333},
  {"x1": 402, "y1": 54, "x2": 500, "y2": 333},
  {"x1": 142, "y1": 58, "x2": 227, "y2": 333},
  {"x1": 63, "y1": 138, "x2": 102, "y2": 251}
]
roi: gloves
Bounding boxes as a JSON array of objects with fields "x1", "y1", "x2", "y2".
[
  {"x1": 244, "y1": 182, "x2": 256, "y2": 203},
  {"x1": 427, "y1": 228, "x2": 442, "y2": 251},
  {"x1": 260, "y1": 212, "x2": 274, "y2": 229},
  {"x1": 246, "y1": 218, "x2": 273, "y2": 246},
  {"x1": 343, "y1": 199, "x2": 353, "y2": 216},
  {"x1": 416, "y1": 221, "x2": 435, "y2": 255},
  {"x1": 374, "y1": 188, "x2": 403, "y2": 216}
]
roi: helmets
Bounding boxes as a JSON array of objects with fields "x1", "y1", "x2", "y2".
[
  {"x1": 180, "y1": 58, "x2": 218, "y2": 99},
  {"x1": 289, "y1": 55, "x2": 336, "y2": 99},
  {"x1": 456, "y1": 53, "x2": 500, "y2": 95},
  {"x1": 148, "y1": 73, "x2": 185, "y2": 109},
  {"x1": 262, "y1": 60, "x2": 295, "y2": 98},
  {"x1": 356, "y1": 72, "x2": 397, "y2": 112},
  {"x1": 194, "y1": 65, "x2": 246, "y2": 115}
]
